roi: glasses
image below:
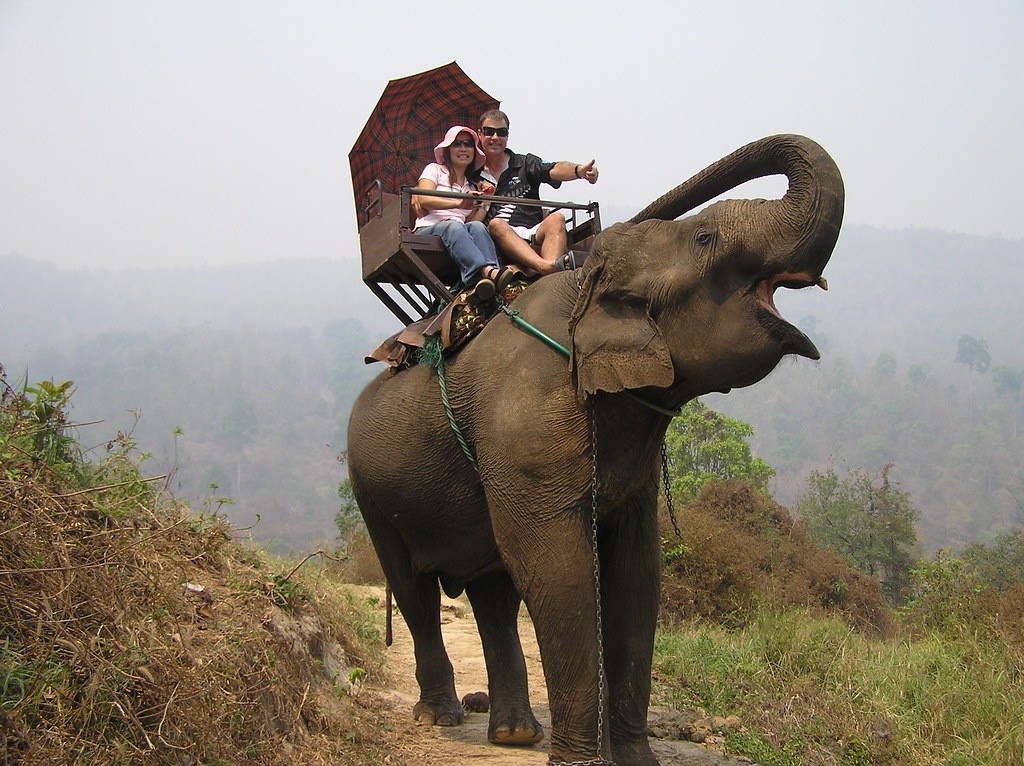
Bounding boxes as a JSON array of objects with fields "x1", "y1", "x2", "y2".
[
  {"x1": 480, "y1": 126, "x2": 509, "y2": 137},
  {"x1": 452, "y1": 139, "x2": 474, "y2": 148}
]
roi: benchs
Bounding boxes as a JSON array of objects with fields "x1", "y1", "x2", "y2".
[{"x1": 358, "y1": 176, "x2": 602, "y2": 321}]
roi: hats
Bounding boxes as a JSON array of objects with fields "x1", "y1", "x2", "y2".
[{"x1": 434, "y1": 125, "x2": 486, "y2": 171}]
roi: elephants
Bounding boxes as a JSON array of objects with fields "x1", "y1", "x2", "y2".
[{"x1": 347, "y1": 133, "x2": 846, "y2": 765}]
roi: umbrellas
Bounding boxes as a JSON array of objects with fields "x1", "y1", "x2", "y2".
[{"x1": 347, "y1": 61, "x2": 503, "y2": 234}]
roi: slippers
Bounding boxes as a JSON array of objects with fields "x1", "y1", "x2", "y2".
[{"x1": 555, "y1": 250, "x2": 575, "y2": 271}]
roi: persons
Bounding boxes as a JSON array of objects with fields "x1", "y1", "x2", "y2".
[
  {"x1": 414, "y1": 125, "x2": 514, "y2": 307},
  {"x1": 411, "y1": 110, "x2": 599, "y2": 275}
]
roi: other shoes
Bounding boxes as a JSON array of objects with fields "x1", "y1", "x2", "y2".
[
  {"x1": 487, "y1": 267, "x2": 514, "y2": 289},
  {"x1": 466, "y1": 278, "x2": 495, "y2": 306}
]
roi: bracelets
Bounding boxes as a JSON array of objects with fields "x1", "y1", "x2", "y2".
[{"x1": 575, "y1": 165, "x2": 583, "y2": 179}]
